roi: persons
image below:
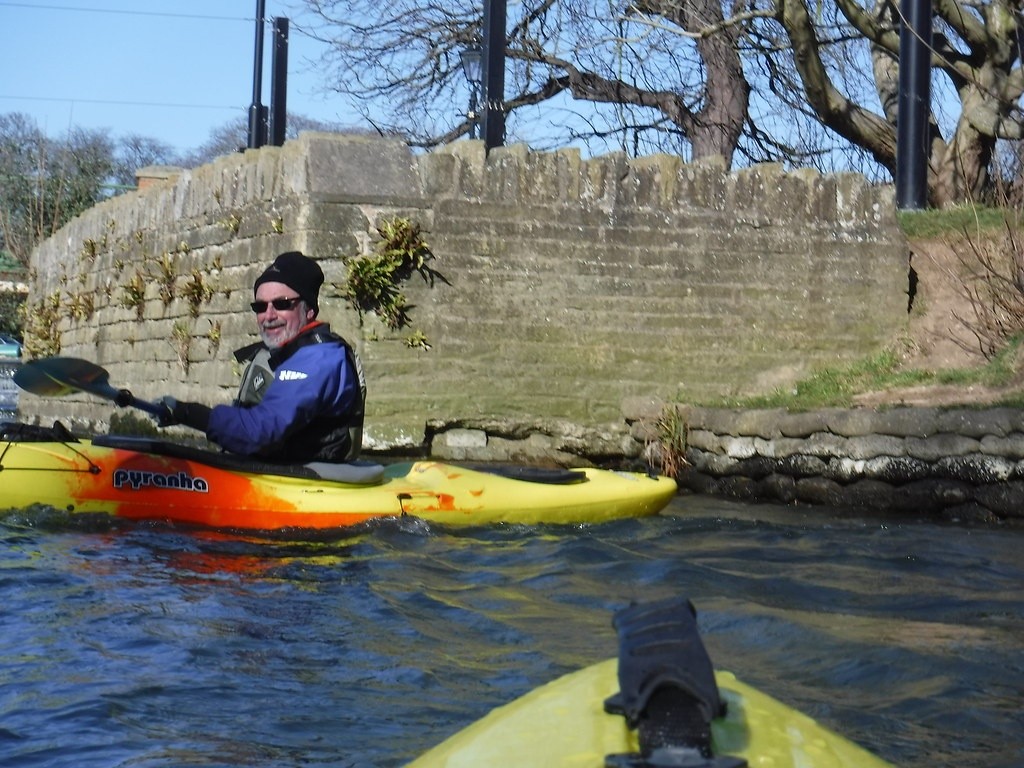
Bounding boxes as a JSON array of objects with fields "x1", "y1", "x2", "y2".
[{"x1": 149, "y1": 250, "x2": 366, "y2": 465}]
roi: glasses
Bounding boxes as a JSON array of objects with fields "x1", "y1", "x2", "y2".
[{"x1": 251, "y1": 296, "x2": 304, "y2": 313}]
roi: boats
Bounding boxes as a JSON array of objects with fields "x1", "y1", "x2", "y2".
[
  {"x1": 0, "y1": 419, "x2": 677, "y2": 532},
  {"x1": 0, "y1": 334, "x2": 23, "y2": 358}
]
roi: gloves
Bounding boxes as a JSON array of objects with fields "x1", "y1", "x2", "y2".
[{"x1": 150, "y1": 396, "x2": 187, "y2": 427}]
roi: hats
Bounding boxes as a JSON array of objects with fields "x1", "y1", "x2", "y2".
[{"x1": 254, "y1": 252, "x2": 325, "y2": 322}]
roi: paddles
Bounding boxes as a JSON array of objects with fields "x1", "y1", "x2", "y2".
[{"x1": 12, "y1": 356, "x2": 207, "y2": 433}]
